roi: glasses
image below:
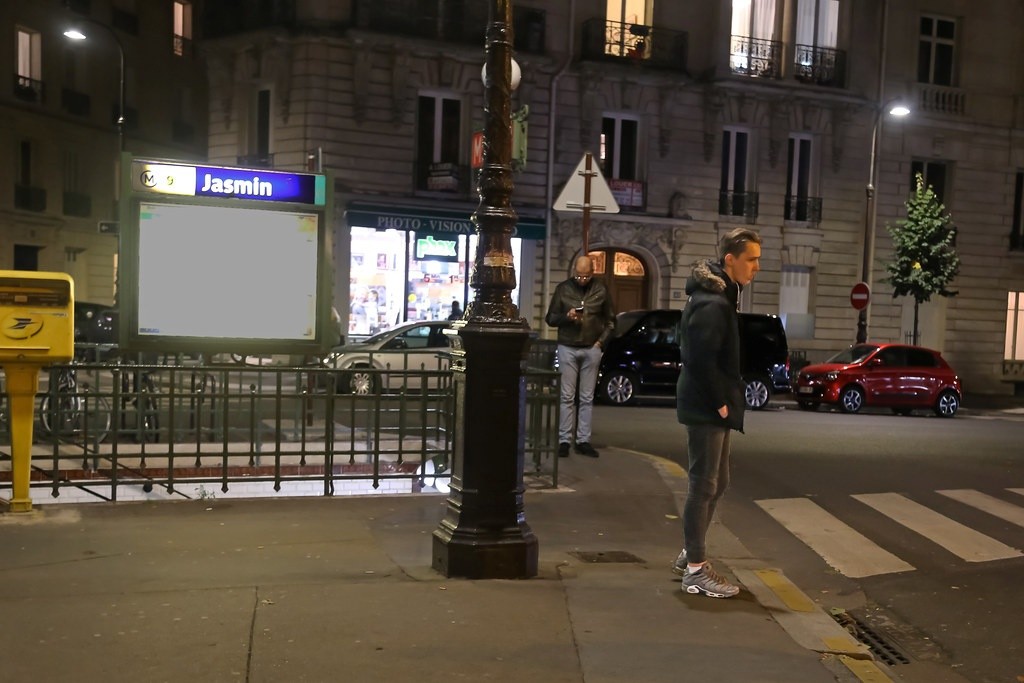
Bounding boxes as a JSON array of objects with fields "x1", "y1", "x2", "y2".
[{"x1": 574, "y1": 270, "x2": 593, "y2": 281}]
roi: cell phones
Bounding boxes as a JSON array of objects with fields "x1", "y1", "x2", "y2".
[{"x1": 574, "y1": 307, "x2": 584, "y2": 317}]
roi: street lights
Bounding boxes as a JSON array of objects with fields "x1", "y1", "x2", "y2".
[
  {"x1": 856, "y1": 94, "x2": 907, "y2": 343},
  {"x1": 64, "y1": 25, "x2": 132, "y2": 400}
]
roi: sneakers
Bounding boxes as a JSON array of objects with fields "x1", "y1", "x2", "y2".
[
  {"x1": 558, "y1": 442, "x2": 570, "y2": 457},
  {"x1": 574, "y1": 442, "x2": 599, "y2": 457},
  {"x1": 671, "y1": 549, "x2": 740, "y2": 598}
]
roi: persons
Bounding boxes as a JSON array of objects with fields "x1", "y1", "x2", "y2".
[
  {"x1": 545, "y1": 255, "x2": 613, "y2": 459},
  {"x1": 670, "y1": 227, "x2": 765, "y2": 599},
  {"x1": 446, "y1": 302, "x2": 463, "y2": 321}
]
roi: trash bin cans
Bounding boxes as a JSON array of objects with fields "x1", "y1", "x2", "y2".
[{"x1": 787, "y1": 349, "x2": 811, "y2": 393}]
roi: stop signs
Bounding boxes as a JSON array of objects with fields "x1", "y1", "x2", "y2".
[{"x1": 850, "y1": 282, "x2": 871, "y2": 310}]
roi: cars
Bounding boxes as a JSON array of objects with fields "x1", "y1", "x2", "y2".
[
  {"x1": 72, "y1": 301, "x2": 119, "y2": 366},
  {"x1": 324, "y1": 318, "x2": 468, "y2": 398},
  {"x1": 787, "y1": 342, "x2": 962, "y2": 417}
]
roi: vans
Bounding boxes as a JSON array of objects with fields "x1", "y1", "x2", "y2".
[{"x1": 552, "y1": 309, "x2": 791, "y2": 409}]
